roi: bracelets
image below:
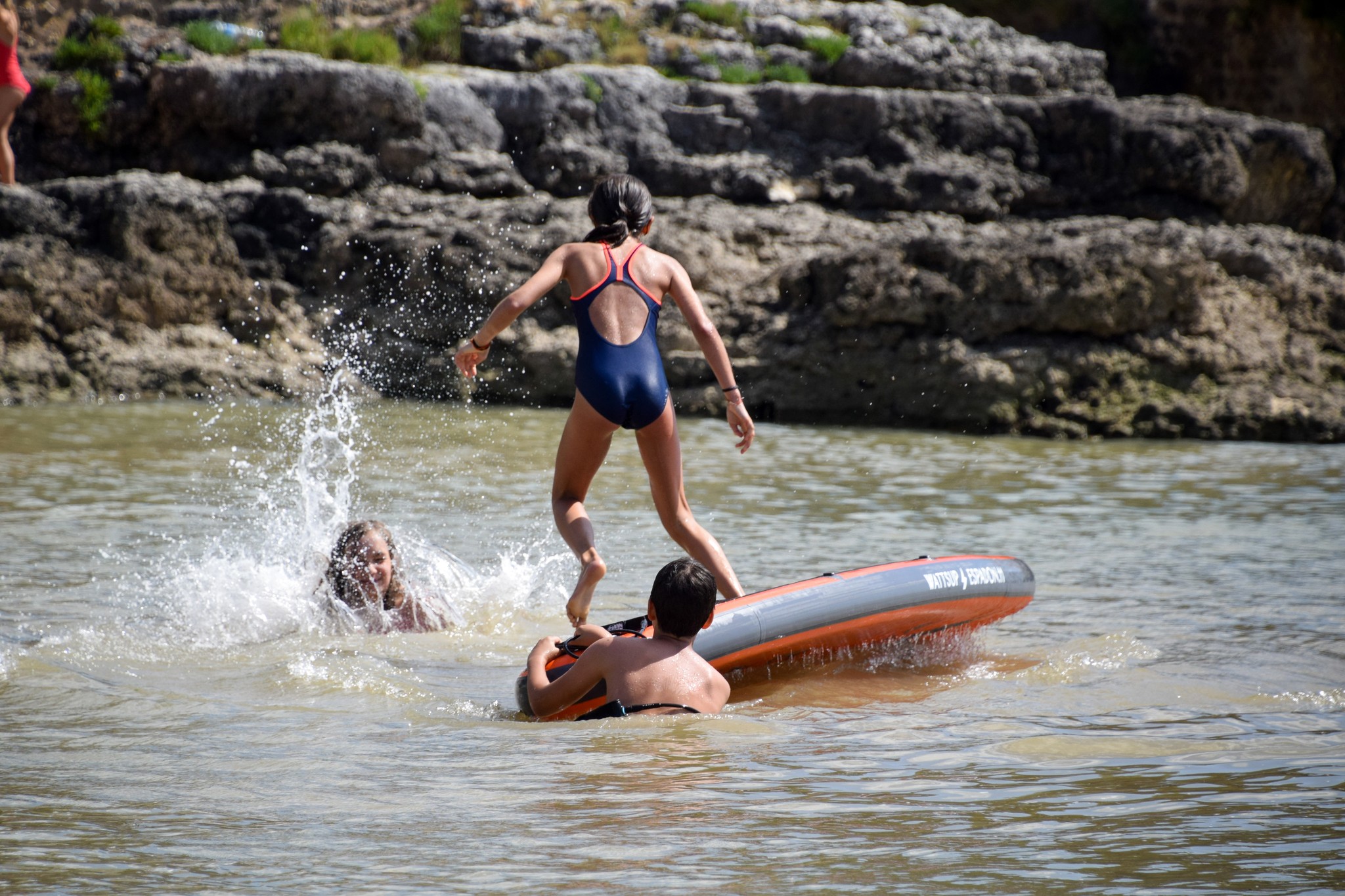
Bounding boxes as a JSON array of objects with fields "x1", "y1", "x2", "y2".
[
  {"x1": 726, "y1": 396, "x2": 744, "y2": 404},
  {"x1": 721, "y1": 385, "x2": 739, "y2": 393}
]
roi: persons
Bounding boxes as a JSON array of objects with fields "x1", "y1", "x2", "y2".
[
  {"x1": 311, "y1": 520, "x2": 455, "y2": 634},
  {"x1": 527, "y1": 558, "x2": 731, "y2": 717},
  {"x1": 456, "y1": 176, "x2": 755, "y2": 625}
]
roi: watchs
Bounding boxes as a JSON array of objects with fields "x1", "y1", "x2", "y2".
[{"x1": 470, "y1": 336, "x2": 493, "y2": 351}]
